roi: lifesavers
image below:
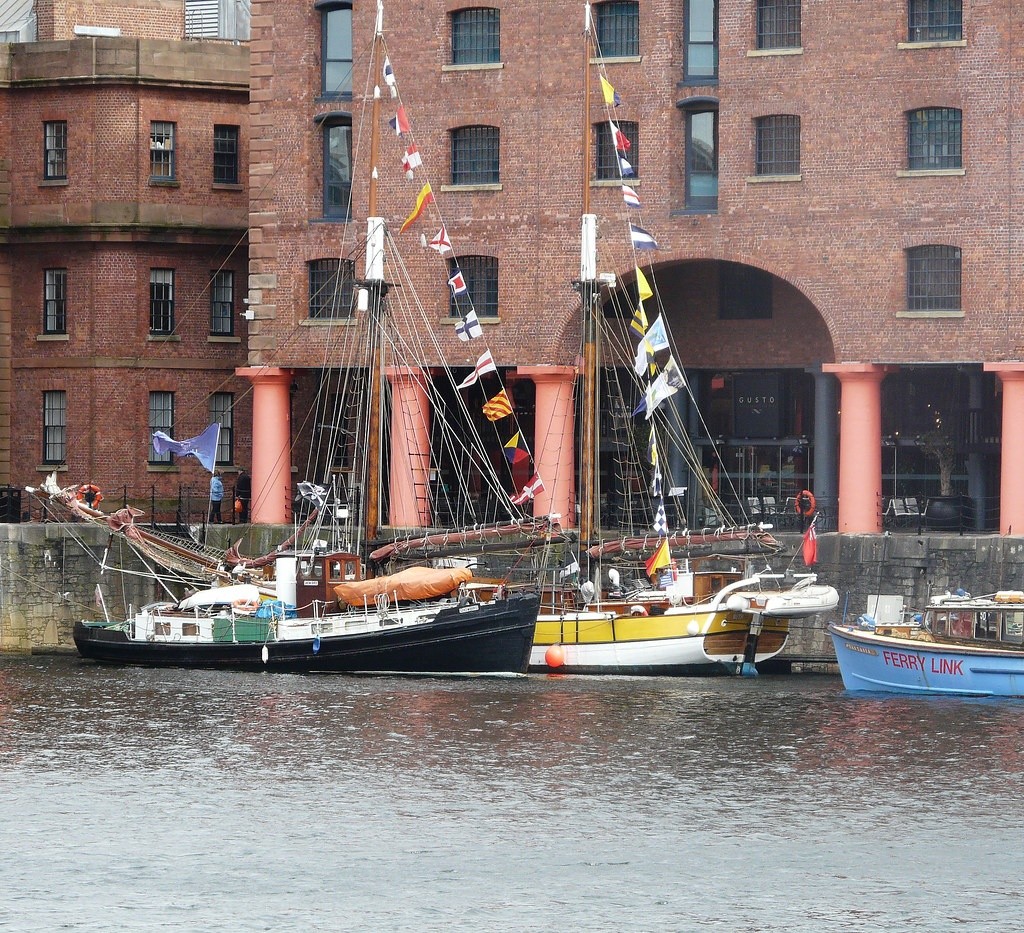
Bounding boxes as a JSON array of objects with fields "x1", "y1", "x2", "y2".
[
  {"x1": 234, "y1": 598, "x2": 258, "y2": 611},
  {"x1": 630, "y1": 605, "x2": 649, "y2": 617},
  {"x1": 794, "y1": 490, "x2": 816, "y2": 515},
  {"x1": 77, "y1": 485, "x2": 101, "y2": 509}
]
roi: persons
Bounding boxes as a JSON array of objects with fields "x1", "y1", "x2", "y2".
[
  {"x1": 235, "y1": 467, "x2": 251, "y2": 525},
  {"x1": 207, "y1": 467, "x2": 224, "y2": 526}
]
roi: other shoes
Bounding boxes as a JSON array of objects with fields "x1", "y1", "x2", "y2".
[
  {"x1": 209, "y1": 521, "x2": 215, "y2": 524},
  {"x1": 238, "y1": 520, "x2": 243, "y2": 524},
  {"x1": 218, "y1": 520, "x2": 225, "y2": 524},
  {"x1": 243, "y1": 520, "x2": 247, "y2": 523}
]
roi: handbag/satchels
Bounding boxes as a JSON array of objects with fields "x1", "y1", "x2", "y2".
[{"x1": 235, "y1": 499, "x2": 242, "y2": 512}]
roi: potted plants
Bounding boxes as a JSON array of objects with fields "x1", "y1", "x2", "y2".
[{"x1": 925, "y1": 408, "x2": 970, "y2": 530}]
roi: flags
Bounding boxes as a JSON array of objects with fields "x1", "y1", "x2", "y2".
[
  {"x1": 95, "y1": 587, "x2": 101, "y2": 607},
  {"x1": 599, "y1": 77, "x2": 688, "y2": 587},
  {"x1": 382, "y1": 57, "x2": 545, "y2": 505},
  {"x1": 802, "y1": 522, "x2": 822, "y2": 567},
  {"x1": 153, "y1": 423, "x2": 220, "y2": 472}
]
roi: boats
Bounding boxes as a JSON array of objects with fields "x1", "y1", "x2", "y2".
[{"x1": 827, "y1": 588, "x2": 1024, "y2": 697}]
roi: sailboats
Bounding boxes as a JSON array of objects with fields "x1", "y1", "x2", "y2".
[{"x1": 25, "y1": 0, "x2": 840, "y2": 677}]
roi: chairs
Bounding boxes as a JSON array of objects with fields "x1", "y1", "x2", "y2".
[
  {"x1": 883, "y1": 498, "x2": 921, "y2": 528},
  {"x1": 748, "y1": 495, "x2": 837, "y2": 532}
]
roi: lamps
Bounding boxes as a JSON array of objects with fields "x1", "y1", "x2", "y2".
[
  {"x1": 716, "y1": 435, "x2": 727, "y2": 445},
  {"x1": 914, "y1": 434, "x2": 926, "y2": 447},
  {"x1": 291, "y1": 378, "x2": 299, "y2": 394},
  {"x1": 885, "y1": 435, "x2": 896, "y2": 446},
  {"x1": 798, "y1": 434, "x2": 810, "y2": 444}
]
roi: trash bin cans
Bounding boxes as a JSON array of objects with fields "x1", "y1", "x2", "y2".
[{"x1": 0, "y1": 488, "x2": 22, "y2": 524}]
